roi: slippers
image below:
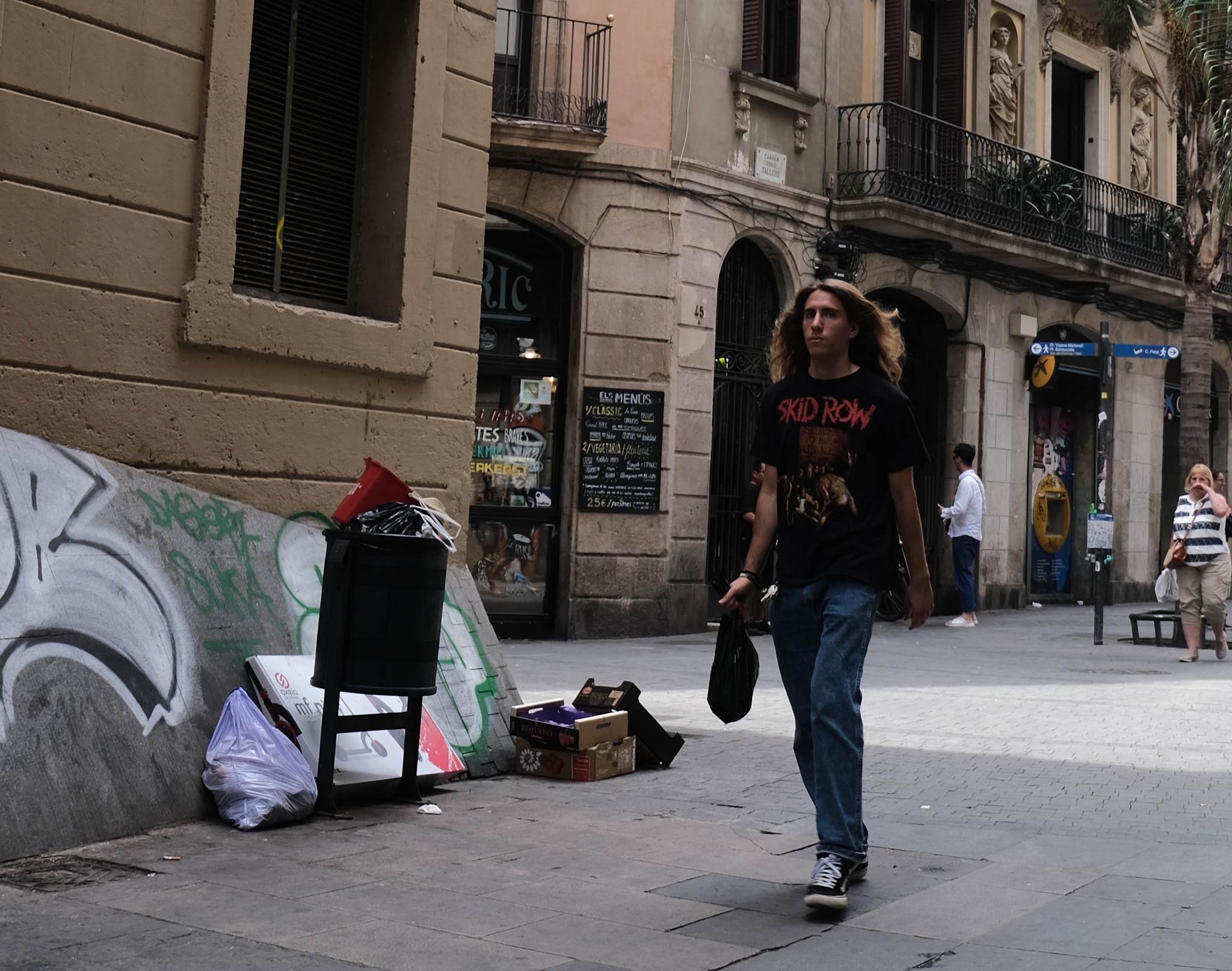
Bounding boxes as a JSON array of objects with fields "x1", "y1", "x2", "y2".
[{"x1": 944, "y1": 615, "x2": 978, "y2": 628}]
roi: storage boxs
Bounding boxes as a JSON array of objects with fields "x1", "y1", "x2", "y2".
[{"x1": 508, "y1": 677, "x2": 686, "y2": 783}]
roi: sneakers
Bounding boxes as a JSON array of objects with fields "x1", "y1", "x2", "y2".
[{"x1": 803, "y1": 851, "x2": 868, "y2": 909}]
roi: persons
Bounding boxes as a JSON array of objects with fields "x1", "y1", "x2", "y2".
[
  {"x1": 715, "y1": 276, "x2": 935, "y2": 911},
  {"x1": 1172, "y1": 464, "x2": 1232, "y2": 663},
  {"x1": 739, "y1": 442, "x2": 985, "y2": 629}
]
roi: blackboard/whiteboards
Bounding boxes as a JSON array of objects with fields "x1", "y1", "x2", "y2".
[{"x1": 578, "y1": 386, "x2": 665, "y2": 514}]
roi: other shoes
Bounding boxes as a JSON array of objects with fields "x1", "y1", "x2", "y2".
[
  {"x1": 1215, "y1": 631, "x2": 1228, "y2": 659},
  {"x1": 1178, "y1": 650, "x2": 1199, "y2": 662}
]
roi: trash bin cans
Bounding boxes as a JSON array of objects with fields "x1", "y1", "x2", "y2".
[{"x1": 311, "y1": 527, "x2": 454, "y2": 696}]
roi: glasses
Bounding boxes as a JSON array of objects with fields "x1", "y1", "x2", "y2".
[
  {"x1": 1216, "y1": 478, "x2": 1223, "y2": 482},
  {"x1": 953, "y1": 456, "x2": 957, "y2": 460}
]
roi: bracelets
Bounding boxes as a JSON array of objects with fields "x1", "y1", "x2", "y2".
[
  {"x1": 741, "y1": 571, "x2": 758, "y2": 579},
  {"x1": 739, "y1": 571, "x2": 756, "y2": 585}
]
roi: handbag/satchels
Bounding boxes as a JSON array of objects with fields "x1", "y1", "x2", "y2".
[
  {"x1": 707, "y1": 575, "x2": 760, "y2": 724},
  {"x1": 1163, "y1": 539, "x2": 1186, "y2": 568},
  {"x1": 1154, "y1": 568, "x2": 1179, "y2": 603},
  {"x1": 944, "y1": 500, "x2": 956, "y2": 533}
]
